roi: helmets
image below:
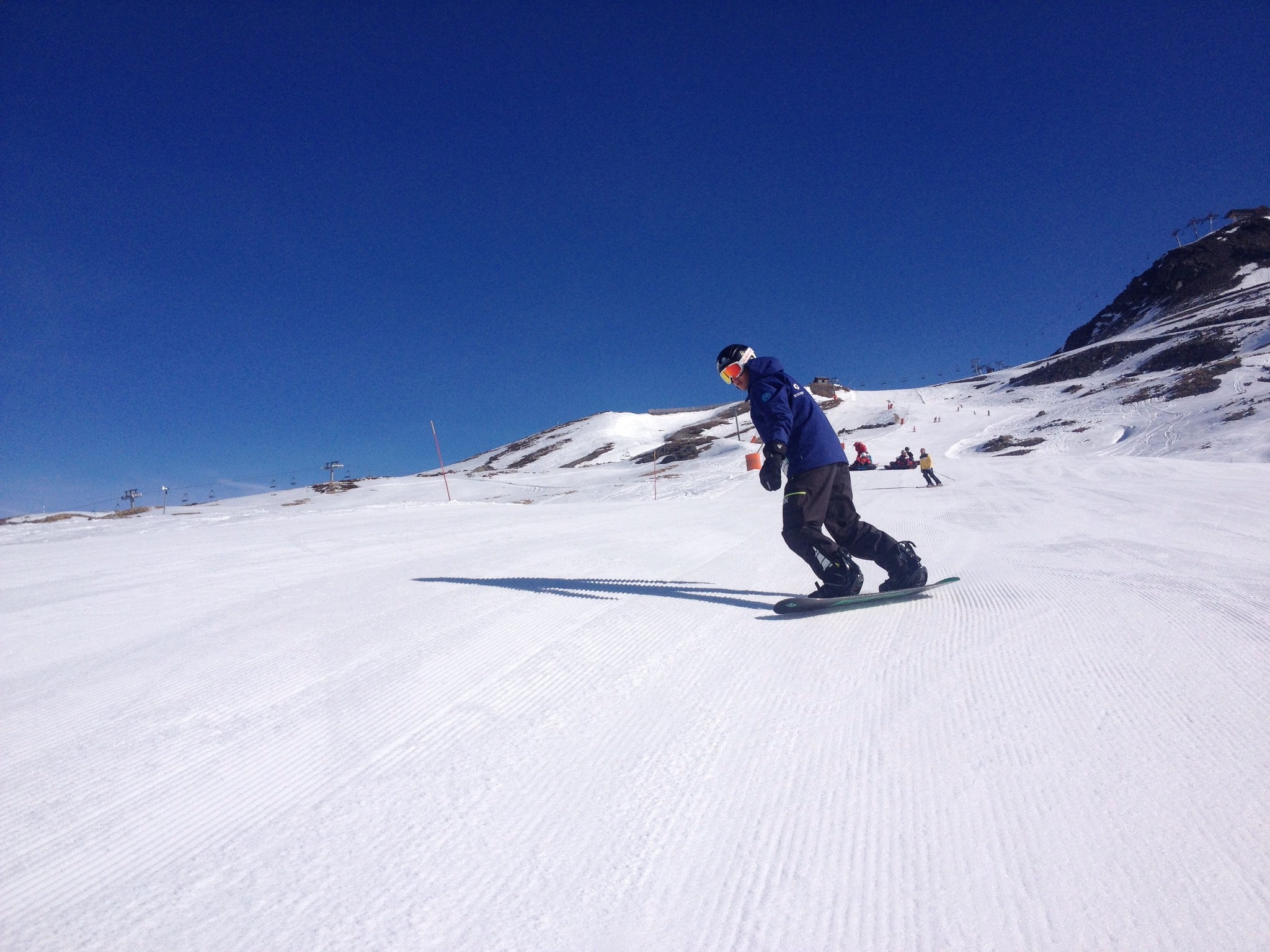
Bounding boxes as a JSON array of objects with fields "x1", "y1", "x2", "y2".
[
  {"x1": 716, "y1": 343, "x2": 757, "y2": 385},
  {"x1": 905, "y1": 447, "x2": 910, "y2": 451},
  {"x1": 901, "y1": 450, "x2": 906, "y2": 455}
]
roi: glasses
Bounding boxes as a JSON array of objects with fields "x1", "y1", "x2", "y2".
[{"x1": 720, "y1": 361, "x2": 744, "y2": 384}]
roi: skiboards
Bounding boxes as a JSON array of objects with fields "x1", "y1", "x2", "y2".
[{"x1": 916, "y1": 481, "x2": 949, "y2": 488}]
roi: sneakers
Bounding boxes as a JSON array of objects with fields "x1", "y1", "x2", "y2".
[
  {"x1": 808, "y1": 546, "x2": 864, "y2": 598},
  {"x1": 936, "y1": 481, "x2": 942, "y2": 486},
  {"x1": 926, "y1": 483, "x2": 935, "y2": 487},
  {"x1": 877, "y1": 539, "x2": 929, "y2": 591}
]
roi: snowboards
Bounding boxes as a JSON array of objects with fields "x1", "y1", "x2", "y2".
[
  {"x1": 773, "y1": 576, "x2": 961, "y2": 615},
  {"x1": 849, "y1": 463, "x2": 880, "y2": 468},
  {"x1": 884, "y1": 464, "x2": 917, "y2": 469},
  {"x1": 889, "y1": 460, "x2": 920, "y2": 466},
  {"x1": 848, "y1": 465, "x2": 877, "y2": 471}
]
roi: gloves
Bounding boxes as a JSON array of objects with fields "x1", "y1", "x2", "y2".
[
  {"x1": 897, "y1": 458, "x2": 900, "y2": 461},
  {"x1": 759, "y1": 440, "x2": 788, "y2": 491},
  {"x1": 903, "y1": 458, "x2": 905, "y2": 460},
  {"x1": 928, "y1": 467, "x2": 933, "y2": 472},
  {"x1": 921, "y1": 470, "x2": 924, "y2": 473},
  {"x1": 866, "y1": 462, "x2": 868, "y2": 466}
]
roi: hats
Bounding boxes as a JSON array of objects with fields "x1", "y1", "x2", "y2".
[
  {"x1": 865, "y1": 450, "x2": 870, "y2": 453},
  {"x1": 861, "y1": 453, "x2": 867, "y2": 457},
  {"x1": 853, "y1": 442, "x2": 859, "y2": 446}
]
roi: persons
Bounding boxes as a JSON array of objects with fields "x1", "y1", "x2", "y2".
[
  {"x1": 938, "y1": 417, "x2": 940, "y2": 422},
  {"x1": 919, "y1": 448, "x2": 942, "y2": 486},
  {"x1": 974, "y1": 411, "x2": 975, "y2": 414},
  {"x1": 988, "y1": 410, "x2": 990, "y2": 416},
  {"x1": 934, "y1": 417, "x2": 937, "y2": 422},
  {"x1": 849, "y1": 442, "x2": 872, "y2": 466},
  {"x1": 895, "y1": 447, "x2": 914, "y2": 466},
  {"x1": 913, "y1": 426, "x2": 916, "y2": 432},
  {"x1": 717, "y1": 344, "x2": 928, "y2": 596},
  {"x1": 961, "y1": 404, "x2": 963, "y2": 408},
  {"x1": 958, "y1": 407, "x2": 959, "y2": 411}
]
